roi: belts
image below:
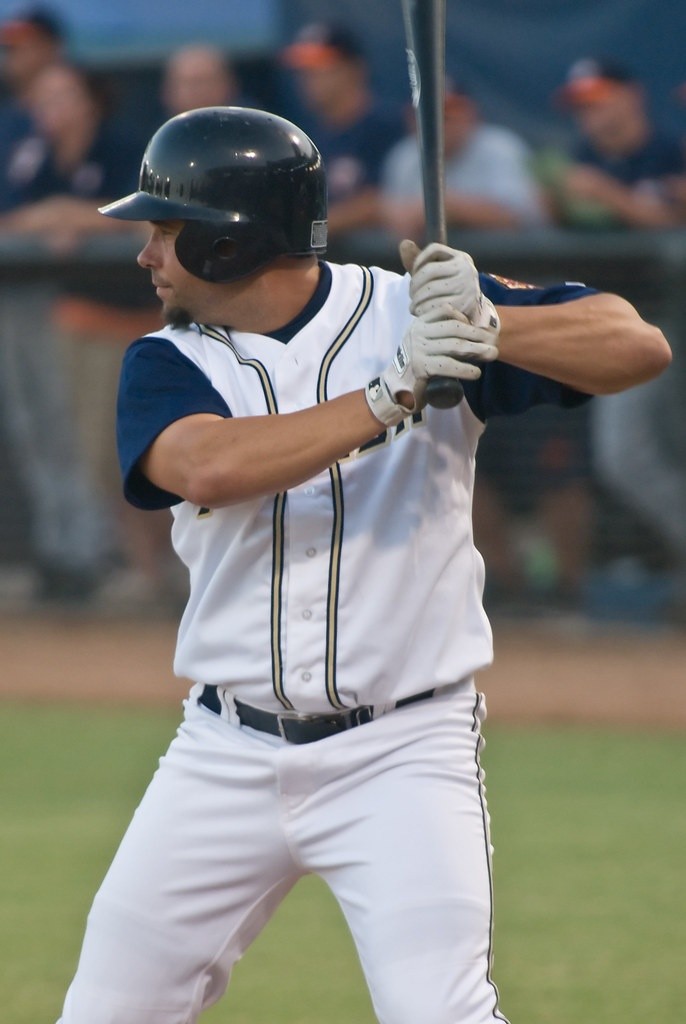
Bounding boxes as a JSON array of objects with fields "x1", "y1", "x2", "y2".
[{"x1": 201, "y1": 684, "x2": 434, "y2": 745}]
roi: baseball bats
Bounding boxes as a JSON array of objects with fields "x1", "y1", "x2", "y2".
[{"x1": 397, "y1": 0, "x2": 470, "y2": 409}]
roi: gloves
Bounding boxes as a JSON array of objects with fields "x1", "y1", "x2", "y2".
[
  {"x1": 398, "y1": 237, "x2": 500, "y2": 346},
  {"x1": 361, "y1": 295, "x2": 499, "y2": 428}
]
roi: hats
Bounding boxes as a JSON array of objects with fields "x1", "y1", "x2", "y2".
[
  {"x1": 286, "y1": 22, "x2": 358, "y2": 70},
  {"x1": 0, "y1": 10, "x2": 58, "y2": 49},
  {"x1": 554, "y1": 59, "x2": 634, "y2": 108}
]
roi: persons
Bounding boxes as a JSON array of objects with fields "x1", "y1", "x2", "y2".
[
  {"x1": 1, "y1": 1, "x2": 686, "y2": 609},
  {"x1": 59, "y1": 106, "x2": 673, "y2": 1024}
]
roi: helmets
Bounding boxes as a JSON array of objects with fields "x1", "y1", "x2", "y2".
[{"x1": 95, "y1": 106, "x2": 330, "y2": 284}]
